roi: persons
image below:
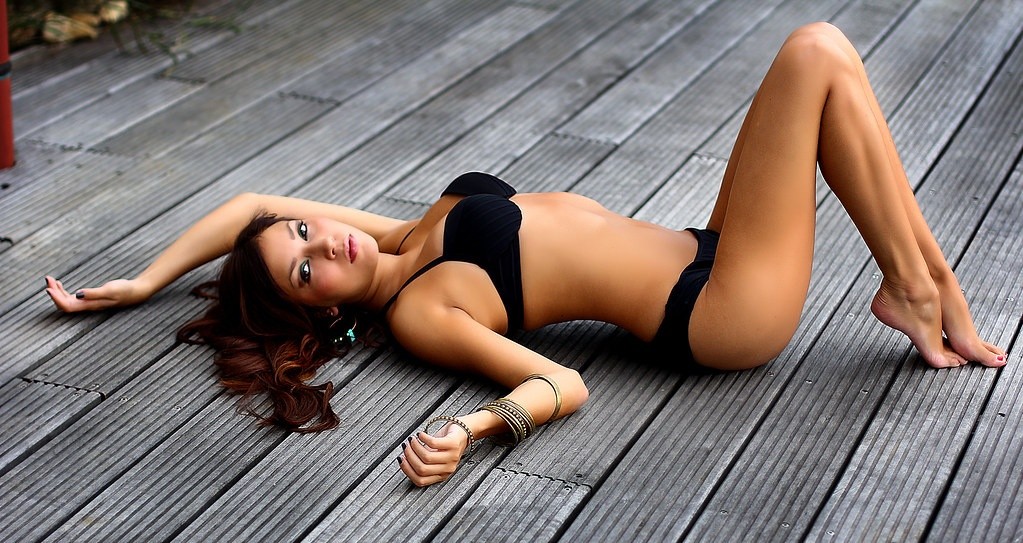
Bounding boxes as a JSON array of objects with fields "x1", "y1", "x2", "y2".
[{"x1": 44, "y1": 21, "x2": 1008, "y2": 487}]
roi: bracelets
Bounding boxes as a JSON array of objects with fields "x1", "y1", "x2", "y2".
[
  {"x1": 423, "y1": 416, "x2": 475, "y2": 460},
  {"x1": 521, "y1": 374, "x2": 562, "y2": 427},
  {"x1": 475, "y1": 398, "x2": 535, "y2": 447}
]
